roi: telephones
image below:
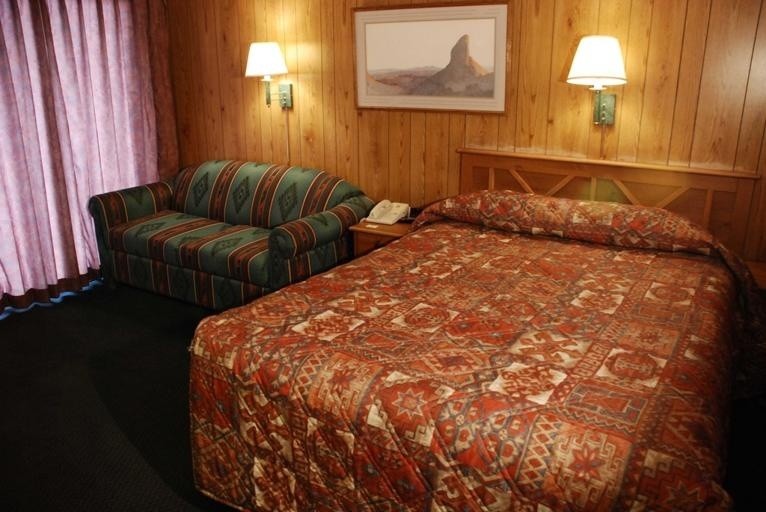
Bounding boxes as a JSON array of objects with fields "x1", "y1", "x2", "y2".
[{"x1": 365, "y1": 199, "x2": 409, "y2": 225}]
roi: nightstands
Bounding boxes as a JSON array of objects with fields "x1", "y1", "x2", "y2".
[{"x1": 351, "y1": 218, "x2": 412, "y2": 256}]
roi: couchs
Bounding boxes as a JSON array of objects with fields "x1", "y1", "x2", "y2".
[{"x1": 85, "y1": 160, "x2": 376, "y2": 312}]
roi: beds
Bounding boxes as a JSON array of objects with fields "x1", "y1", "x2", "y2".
[{"x1": 188, "y1": 144, "x2": 762, "y2": 512}]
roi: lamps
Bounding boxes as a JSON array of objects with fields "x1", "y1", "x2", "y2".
[
  {"x1": 564, "y1": 35, "x2": 630, "y2": 128},
  {"x1": 243, "y1": 41, "x2": 293, "y2": 110}
]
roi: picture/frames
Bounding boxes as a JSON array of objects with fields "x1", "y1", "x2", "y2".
[{"x1": 349, "y1": 3, "x2": 509, "y2": 115}]
